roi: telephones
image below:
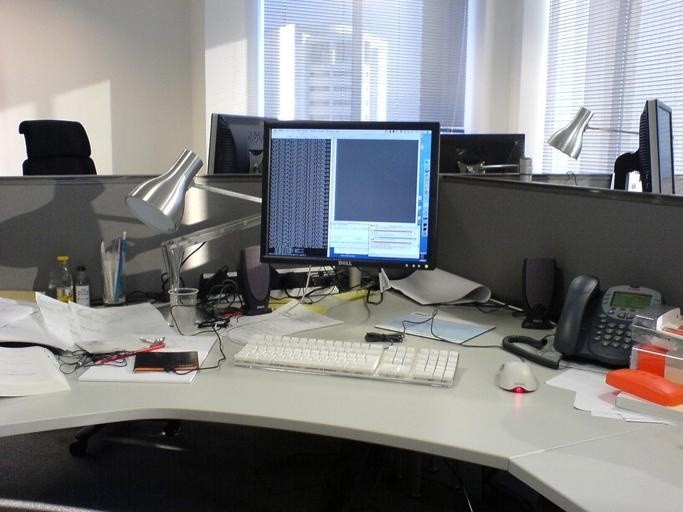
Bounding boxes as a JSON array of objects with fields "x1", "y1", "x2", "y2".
[{"x1": 553, "y1": 273, "x2": 661, "y2": 369}]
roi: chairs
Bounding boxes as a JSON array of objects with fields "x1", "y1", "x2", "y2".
[{"x1": 18, "y1": 120, "x2": 97, "y2": 174}]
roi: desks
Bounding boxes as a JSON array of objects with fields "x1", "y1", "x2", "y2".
[
  {"x1": 507, "y1": 424, "x2": 683, "y2": 511},
  {"x1": 1, "y1": 288, "x2": 683, "y2": 471}
]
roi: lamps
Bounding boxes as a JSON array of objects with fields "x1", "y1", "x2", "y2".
[
  {"x1": 124, "y1": 150, "x2": 262, "y2": 325},
  {"x1": 547, "y1": 108, "x2": 640, "y2": 160}
]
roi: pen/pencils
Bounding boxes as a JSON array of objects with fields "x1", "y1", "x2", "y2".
[
  {"x1": 138, "y1": 336, "x2": 165, "y2": 344},
  {"x1": 100, "y1": 231, "x2": 126, "y2": 302},
  {"x1": 94, "y1": 342, "x2": 165, "y2": 365}
]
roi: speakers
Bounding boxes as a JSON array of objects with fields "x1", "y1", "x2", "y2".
[
  {"x1": 520, "y1": 257, "x2": 557, "y2": 330},
  {"x1": 239, "y1": 246, "x2": 271, "y2": 316}
]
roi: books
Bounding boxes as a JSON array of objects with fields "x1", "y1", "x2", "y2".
[
  {"x1": 79, "y1": 333, "x2": 218, "y2": 383},
  {"x1": 614, "y1": 391, "x2": 683, "y2": 422},
  {"x1": 0, "y1": 346, "x2": 71, "y2": 397}
]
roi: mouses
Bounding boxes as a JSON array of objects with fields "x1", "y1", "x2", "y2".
[{"x1": 495, "y1": 362, "x2": 539, "y2": 393}]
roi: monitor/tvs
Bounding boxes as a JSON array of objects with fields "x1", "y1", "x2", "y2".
[
  {"x1": 614, "y1": 99, "x2": 675, "y2": 196},
  {"x1": 260, "y1": 119, "x2": 441, "y2": 292},
  {"x1": 439, "y1": 132, "x2": 527, "y2": 176},
  {"x1": 205, "y1": 113, "x2": 278, "y2": 175}
]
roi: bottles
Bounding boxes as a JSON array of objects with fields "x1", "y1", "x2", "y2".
[{"x1": 46, "y1": 255, "x2": 92, "y2": 307}]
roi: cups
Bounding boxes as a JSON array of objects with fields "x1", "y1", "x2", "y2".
[{"x1": 167, "y1": 288, "x2": 199, "y2": 333}]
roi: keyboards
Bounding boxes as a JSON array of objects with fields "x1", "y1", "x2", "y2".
[{"x1": 234, "y1": 333, "x2": 460, "y2": 389}]
